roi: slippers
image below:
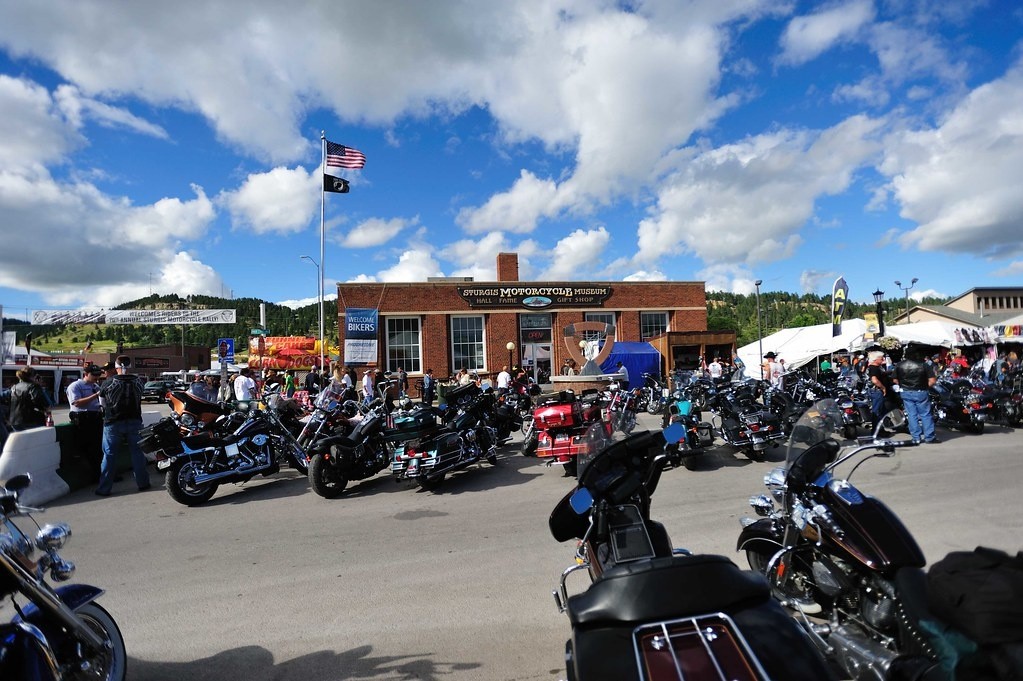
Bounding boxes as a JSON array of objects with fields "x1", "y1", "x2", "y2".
[{"x1": 926, "y1": 437, "x2": 941, "y2": 442}]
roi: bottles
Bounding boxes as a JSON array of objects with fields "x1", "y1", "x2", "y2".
[{"x1": 46, "y1": 415, "x2": 54, "y2": 427}]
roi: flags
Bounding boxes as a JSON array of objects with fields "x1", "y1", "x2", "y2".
[
  {"x1": 327, "y1": 141, "x2": 366, "y2": 169},
  {"x1": 323, "y1": 173, "x2": 349, "y2": 193}
]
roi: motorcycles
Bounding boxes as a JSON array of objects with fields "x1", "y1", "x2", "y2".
[
  {"x1": 737, "y1": 397, "x2": 1023, "y2": 681},
  {"x1": 548, "y1": 408, "x2": 856, "y2": 681},
  {"x1": 132, "y1": 360, "x2": 1023, "y2": 508},
  {"x1": 0, "y1": 471, "x2": 126, "y2": 681}
]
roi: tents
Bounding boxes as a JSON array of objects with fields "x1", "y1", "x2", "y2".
[
  {"x1": 586, "y1": 341, "x2": 662, "y2": 393},
  {"x1": 736, "y1": 314, "x2": 1023, "y2": 382}
]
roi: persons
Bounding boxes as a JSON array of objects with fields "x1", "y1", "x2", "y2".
[{"x1": 0, "y1": 347, "x2": 1014, "y2": 495}]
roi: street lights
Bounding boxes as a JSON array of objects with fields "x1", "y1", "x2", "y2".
[
  {"x1": 872, "y1": 288, "x2": 885, "y2": 337},
  {"x1": 895, "y1": 278, "x2": 918, "y2": 322},
  {"x1": 755, "y1": 279, "x2": 764, "y2": 381},
  {"x1": 578, "y1": 340, "x2": 587, "y2": 357},
  {"x1": 300, "y1": 255, "x2": 321, "y2": 342},
  {"x1": 506, "y1": 341, "x2": 514, "y2": 374}
]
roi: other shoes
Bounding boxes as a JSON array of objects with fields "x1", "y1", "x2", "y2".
[
  {"x1": 137, "y1": 483, "x2": 150, "y2": 491},
  {"x1": 112, "y1": 475, "x2": 123, "y2": 482},
  {"x1": 94, "y1": 488, "x2": 107, "y2": 496}
]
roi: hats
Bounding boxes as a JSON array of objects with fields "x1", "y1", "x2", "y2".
[
  {"x1": 312, "y1": 365, "x2": 316, "y2": 369},
  {"x1": 425, "y1": 369, "x2": 433, "y2": 374},
  {"x1": 764, "y1": 352, "x2": 776, "y2": 359},
  {"x1": 616, "y1": 362, "x2": 621, "y2": 364},
  {"x1": 85, "y1": 364, "x2": 101, "y2": 375}
]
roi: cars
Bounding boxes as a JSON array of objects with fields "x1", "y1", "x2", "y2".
[{"x1": 141, "y1": 379, "x2": 185, "y2": 405}]
